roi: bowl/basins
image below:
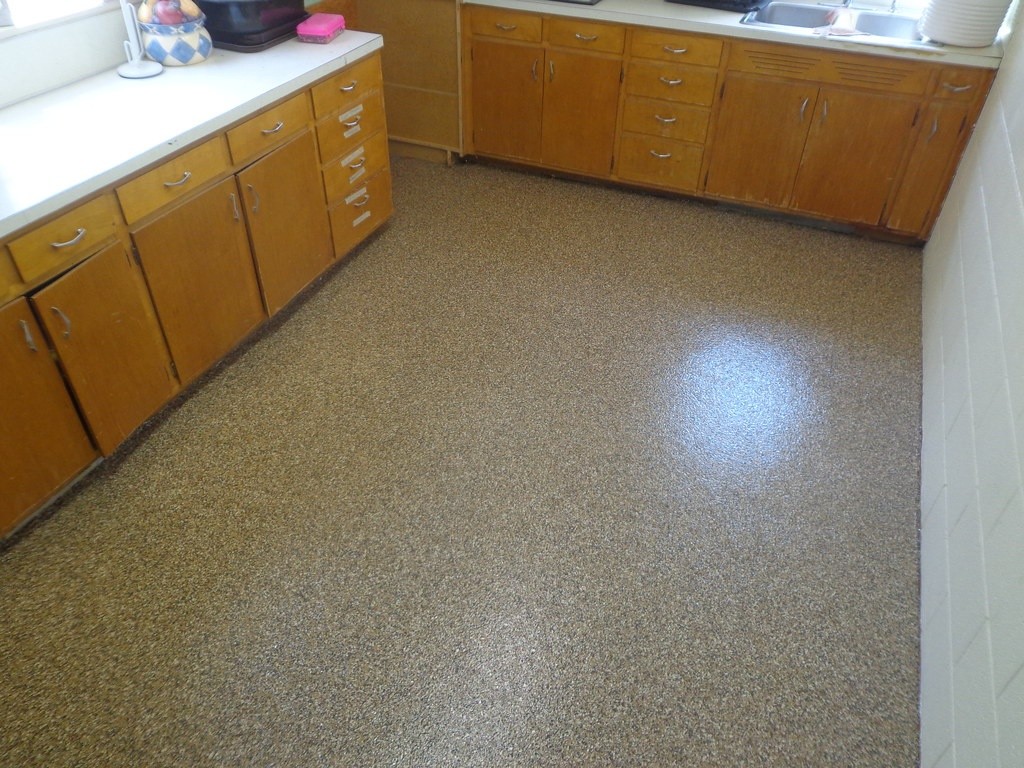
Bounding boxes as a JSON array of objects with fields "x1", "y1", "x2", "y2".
[{"x1": 137, "y1": 12, "x2": 212, "y2": 67}]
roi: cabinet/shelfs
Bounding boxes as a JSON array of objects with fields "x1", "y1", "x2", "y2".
[
  {"x1": 882, "y1": 66, "x2": 1004, "y2": 242},
  {"x1": 610, "y1": 28, "x2": 727, "y2": 198},
  {"x1": 465, "y1": 5, "x2": 626, "y2": 179},
  {"x1": 313, "y1": 1, "x2": 472, "y2": 166},
  {"x1": 0, "y1": 192, "x2": 180, "y2": 546},
  {"x1": 312, "y1": 52, "x2": 395, "y2": 264},
  {"x1": 118, "y1": 88, "x2": 337, "y2": 413},
  {"x1": 700, "y1": 38, "x2": 936, "y2": 227}
]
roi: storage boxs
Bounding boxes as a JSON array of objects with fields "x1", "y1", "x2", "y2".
[{"x1": 296, "y1": 11, "x2": 345, "y2": 43}]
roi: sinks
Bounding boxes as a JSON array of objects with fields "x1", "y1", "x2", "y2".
[
  {"x1": 854, "y1": 11, "x2": 924, "y2": 41},
  {"x1": 753, "y1": 2, "x2": 840, "y2": 29}
]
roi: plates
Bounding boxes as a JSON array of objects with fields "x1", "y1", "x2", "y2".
[{"x1": 922, "y1": 0, "x2": 1012, "y2": 47}]
